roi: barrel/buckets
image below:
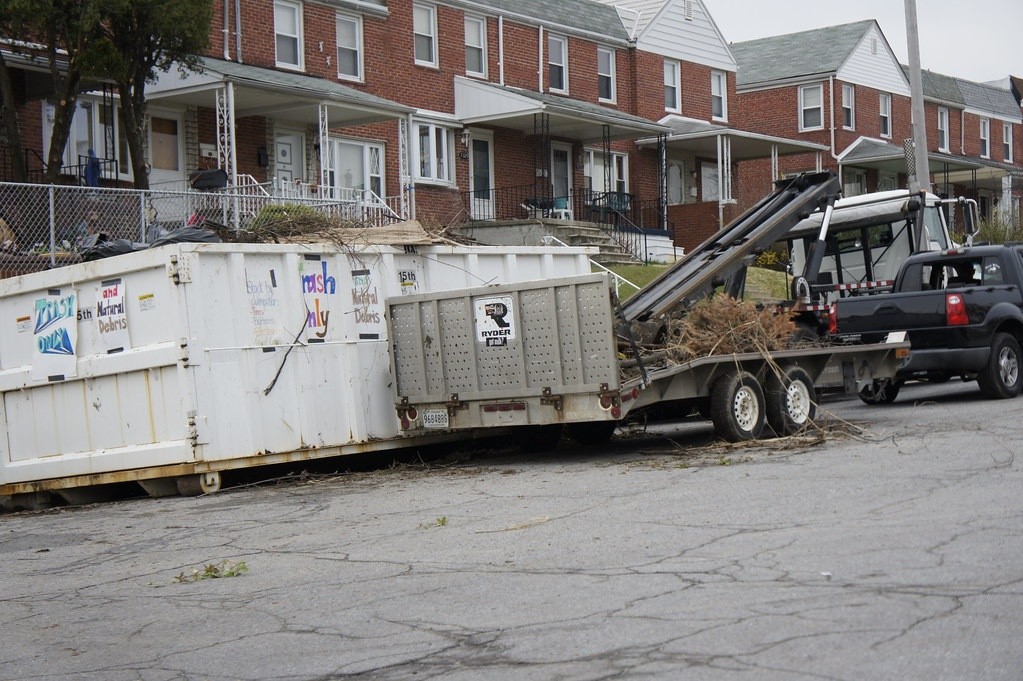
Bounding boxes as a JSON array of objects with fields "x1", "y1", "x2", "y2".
[{"x1": 554, "y1": 197, "x2": 567, "y2": 209}]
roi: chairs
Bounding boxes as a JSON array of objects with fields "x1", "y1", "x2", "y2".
[
  {"x1": 948, "y1": 277, "x2": 974, "y2": 288},
  {"x1": 589, "y1": 192, "x2": 632, "y2": 226},
  {"x1": 880, "y1": 231, "x2": 892, "y2": 246}
]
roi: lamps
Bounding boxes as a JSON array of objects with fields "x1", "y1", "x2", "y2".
[{"x1": 461, "y1": 129, "x2": 472, "y2": 147}]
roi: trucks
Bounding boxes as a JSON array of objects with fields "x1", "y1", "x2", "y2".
[{"x1": 614, "y1": 166, "x2": 981, "y2": 385}]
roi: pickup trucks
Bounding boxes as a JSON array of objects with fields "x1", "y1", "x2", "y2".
[{"x1": 827, "y1": 241, "x2": 1023, "y2": 405}]
